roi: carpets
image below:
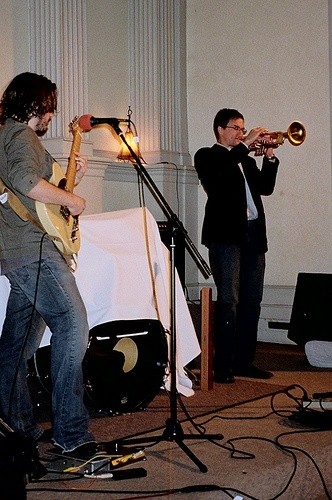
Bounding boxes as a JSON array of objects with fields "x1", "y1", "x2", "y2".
[{"x1": 43, "y1": 376, "x2": 295, "y2": 458}]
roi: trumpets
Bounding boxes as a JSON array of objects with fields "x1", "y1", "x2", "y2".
[{"x1": 234, "y1": 119, "x2": 306, "y2": 156}]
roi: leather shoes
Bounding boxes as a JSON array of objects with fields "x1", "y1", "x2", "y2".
[
  {"x1": 231, "y1": 365, "x2": 273, "y2": 379},
  {"x1": 213, "y1": 355, "x2": 235, "y2": 384}
]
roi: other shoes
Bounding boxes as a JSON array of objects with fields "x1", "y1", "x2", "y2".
[{"x1": 50, "y1": 440, "x2": 107, "y2": 458}]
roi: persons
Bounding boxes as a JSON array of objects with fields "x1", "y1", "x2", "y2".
[
  {"x1": 0, "y1": 70, "x2": 108, "y2": 455},
  {"x1": 194, "y1": 108, "x2": 280, "y2": 384}
]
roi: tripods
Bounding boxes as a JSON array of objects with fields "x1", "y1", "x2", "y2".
[{"x1": 107, "y1": 122, "x2": 225, "y2": 474}]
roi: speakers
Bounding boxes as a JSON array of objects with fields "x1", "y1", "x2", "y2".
[{"x1": 288, "y1": 272, "x2": 332, "y2": 347}]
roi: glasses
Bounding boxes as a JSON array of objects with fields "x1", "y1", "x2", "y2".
[{"x1": 226, "y1": 125, "x2": 247, "y2": 134}]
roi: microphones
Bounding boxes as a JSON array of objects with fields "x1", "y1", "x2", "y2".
[{"x1": 77, "y1": 114, "x2": 131, "y2": 131}]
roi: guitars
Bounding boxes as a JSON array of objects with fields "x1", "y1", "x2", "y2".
[{"x1": 35, "y1": 114, "x2": 87, "y2": 254}]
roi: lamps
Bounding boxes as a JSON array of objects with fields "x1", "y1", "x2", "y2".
[{"x1": 116, "y1": 105, "x2": 141, "y2": 159}]
